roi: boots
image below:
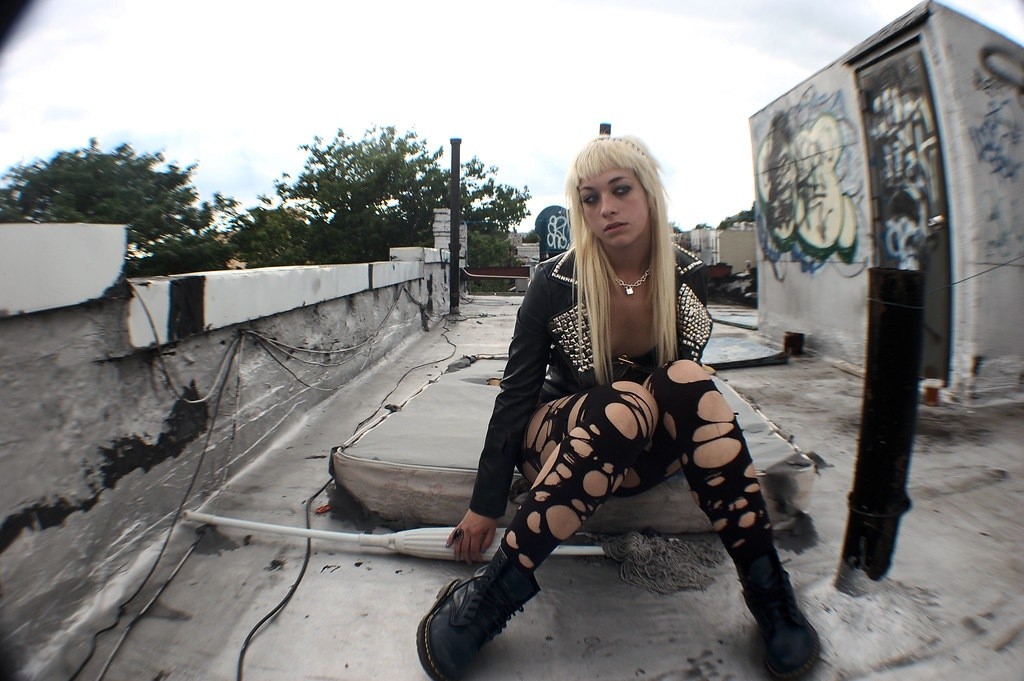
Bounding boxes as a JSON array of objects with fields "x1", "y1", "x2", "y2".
[
  {"x1": 740, "y1": 570, "x2": 822, "y2": 681},
  {"x1": 415, "y1": 545, "x2": 542, "y2": 681}
]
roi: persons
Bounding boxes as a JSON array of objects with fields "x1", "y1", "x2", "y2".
[{"x1": 417, "y1": 134, "x2": 821, "y2": 681}]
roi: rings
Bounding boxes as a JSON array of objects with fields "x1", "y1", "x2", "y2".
[{"x1": 453, "y1": 528, "x2": 463, "y2": 541}]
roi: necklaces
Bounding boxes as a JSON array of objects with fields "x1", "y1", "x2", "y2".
[{"x1": 613, "y1": 265, "x2": 652, "y2": 295}]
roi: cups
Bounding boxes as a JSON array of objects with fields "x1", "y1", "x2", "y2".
[{"x1": 921, "y1": 379, "x2": 945, "y2": 407}]
